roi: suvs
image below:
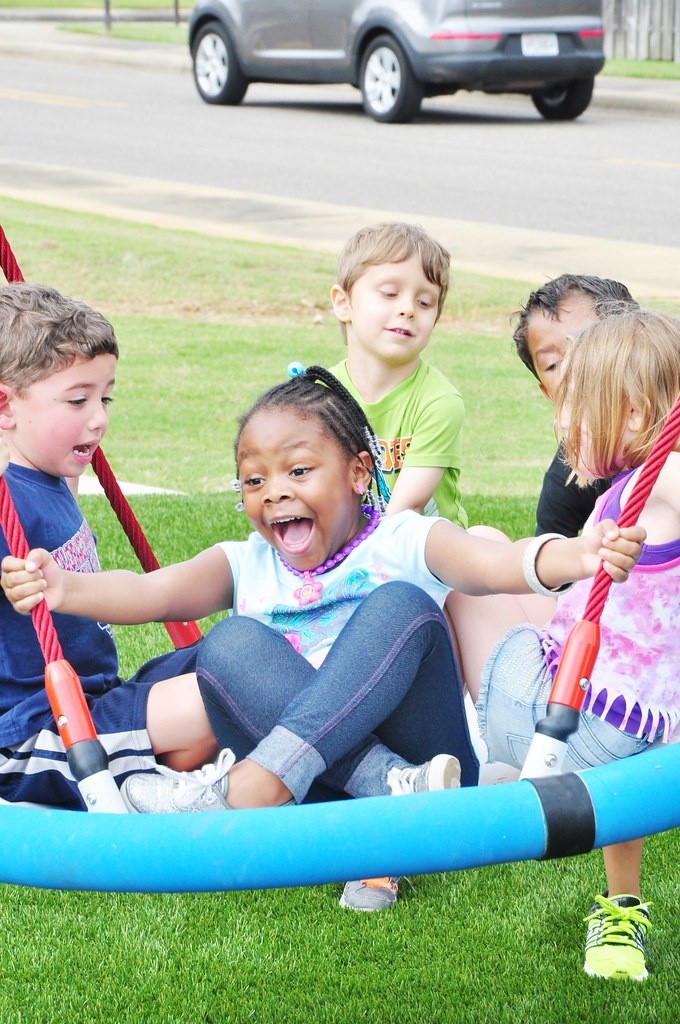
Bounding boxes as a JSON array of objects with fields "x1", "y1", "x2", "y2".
[{"x1": 187, "y1": 0, "x2": 605, "y2": 123}]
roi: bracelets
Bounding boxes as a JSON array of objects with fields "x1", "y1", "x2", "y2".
[{"x1": 522, "y1": 531, "x2": 578, "y2": 596}]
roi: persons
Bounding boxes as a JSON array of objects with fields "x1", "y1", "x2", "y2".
[
  {"x1": 325, "y1": 220, "x2": 467, "y2": 913},
  {"x1": 512, "y1": 276, "x2": 650, "y2": 983},
  {"x1": 0, "y1": 365, "x2": 648, "y2": 822},
  {"x1": 434, "y1": 306, "x2": 679, "y2": 784},
  {"x1": 0, "y1": 282, "x2": 219, "y2": 813}
]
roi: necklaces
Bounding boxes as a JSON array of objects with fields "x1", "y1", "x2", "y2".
[{"x1": 273, "y1": 504, "x2": 381, "y2": 609}]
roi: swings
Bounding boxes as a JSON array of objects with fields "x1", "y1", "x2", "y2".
[{"x1": 1, "y1": 236, "x2": 680, "y2": 895}]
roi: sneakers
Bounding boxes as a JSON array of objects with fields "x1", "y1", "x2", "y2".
[
  {"x1": 583, "y1": 891, "x2": 653, "y2": 983},
  {"x1": 339, "y1": 877, "x2": 401, "y2": 912},
  {"x1": 121, "y1": 748, "x2": 297, "y2": 813},
  {"x1": 386, "y1": 754, "x2": 461, "y2": 796}
]
